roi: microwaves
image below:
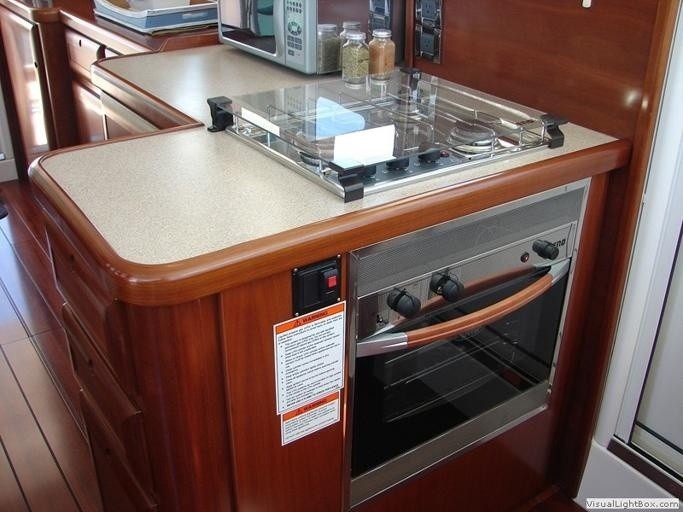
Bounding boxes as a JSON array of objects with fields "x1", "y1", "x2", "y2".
[{"x1": 217, "y1": 0, "x2": 368, "y2": 74}]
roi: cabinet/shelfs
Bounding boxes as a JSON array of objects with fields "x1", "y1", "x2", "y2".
[
  {"x1": 0, "y1": 1, "x2": 53, "y2": 169},
  {"x1": 90, "y1": 64, "x2": 190, "y2": 140},
  {"x1": 61, "y1": 8, "x2": 156, "y2": 141},
  {"x1": 24, "y1": 174, "x2": 163, "y2": 512}
]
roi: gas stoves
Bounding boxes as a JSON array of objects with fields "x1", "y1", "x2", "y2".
[{"x1": 206, "y1": 66, "x2": 568, "y2": 202}]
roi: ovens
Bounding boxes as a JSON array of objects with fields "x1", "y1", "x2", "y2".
[{"x1": 345, "y1": 176, "x2": 592, "y2": 512}]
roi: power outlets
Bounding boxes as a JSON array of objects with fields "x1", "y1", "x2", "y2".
[
  {"x1": 415, "y1": 24, "x2": 442, "y2": 65},
  {"x1": 416, "y1": 0, "x2": 442, "y2": 26}
]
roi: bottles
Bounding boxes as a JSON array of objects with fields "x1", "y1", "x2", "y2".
[
  {"x1": 369, "y1": 29, "x2": 395, "y2": 80},
  {"x1": 342, "y1": 31, "x2": 369, "y2": 84},
  {"x1": 317, "y1": 24, "x2": 342, "y2": 73},
  {"x1": 340, "y1": 22, "x2": 361, "y2": 43}
]
